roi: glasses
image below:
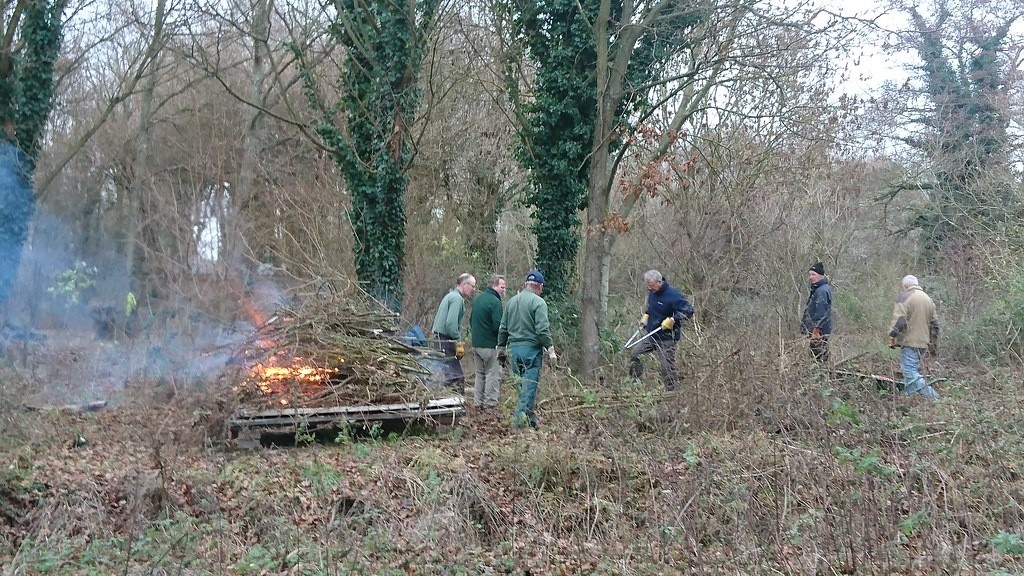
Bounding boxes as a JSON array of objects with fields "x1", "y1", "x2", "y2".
[{"x1": 466, "y1": 282, "x2": 476, "y2": 289}]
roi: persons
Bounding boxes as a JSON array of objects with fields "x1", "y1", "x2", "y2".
[
  {"x1": 628, "y1": 270, "x2": 694, "y2": 391},
  {"x1": 432, "y1": 273, "x2": 476, "y2": 396},
  {"x1": 888, "y1": 275, "x2": 940, "y2": 398},
  {"x1": 800, "y1": 262, "x2": 832, "y2": 361},
  {"x1": 469, "y1": 273, "x2": 507, "y2": 413},
  {"x1": 496, "y1": 271, "x2": 557, "y2": 428}
]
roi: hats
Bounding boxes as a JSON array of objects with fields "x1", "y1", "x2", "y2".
[
  {"x1": 525, "y1": 270, "x2": 546, "y2": 287},
  {"x1": 808, "y1": 261, "x2": 825, "y2": 275}
]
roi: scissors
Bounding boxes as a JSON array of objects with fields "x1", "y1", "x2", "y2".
[{"x1": 617, "y1": 317, "x2": 675, "y2": 354}]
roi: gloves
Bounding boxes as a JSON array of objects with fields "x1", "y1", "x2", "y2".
[
  {"x1": 888, "y1": 337, "x2": 896, "y2": 349},
  {"x1": 454, "y1": 341, "x2": 465, "y2": 360},
  {"x1": 931, "y1": 350, "x2": 937, "y2": 357},
  {"x1": 543, "y1": 345, "x2": 557, "y2": 369},
  {"x1": 810, "y1": 329, "x2": 820, "y2": 339},
  {"x1": 496, "y1": 345, "x2": 510, "y2": 368}
]
